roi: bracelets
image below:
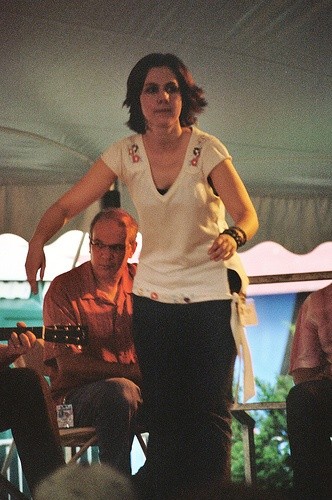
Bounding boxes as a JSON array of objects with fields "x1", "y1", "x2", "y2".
[
  {"x1": 231, "y1": 226, "x2": 247, "y2": 244},
  {"x1": 222, "y1": 229, "x2": 242, "y2": 249}
]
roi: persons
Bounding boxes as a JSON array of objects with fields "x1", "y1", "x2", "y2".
[
  {"x1": 43, "y1": 208, "x2": 148, "y2": 477},
  {"x1": 26, "y1": 52, "x2": 258, "y2": 500},
  {"x1": 286, "y1": 283, "x2": 332, "y2": 500},
  {"x1": 35, "y1": 463, "x2": 135, "y2": 500},
  {"x1": 0, "y1": 323, "x2": 67, "y2": 494}
]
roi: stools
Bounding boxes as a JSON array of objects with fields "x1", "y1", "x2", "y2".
[{"x1": 58, "y1": 425, "x2": 150, "y2": 468}]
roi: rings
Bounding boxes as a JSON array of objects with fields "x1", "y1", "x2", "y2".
[{"x1": 15, "y1": 345, "x2": 20, "y2": 348}]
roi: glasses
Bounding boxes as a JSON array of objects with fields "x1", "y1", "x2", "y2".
[{"x1": 89, "y1": 237, "x2": 123, "y2": 250}]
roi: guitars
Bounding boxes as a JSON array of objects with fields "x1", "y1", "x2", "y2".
[{"x1": 0, "y1": 320, "x2": 90, "y2": 350}]
